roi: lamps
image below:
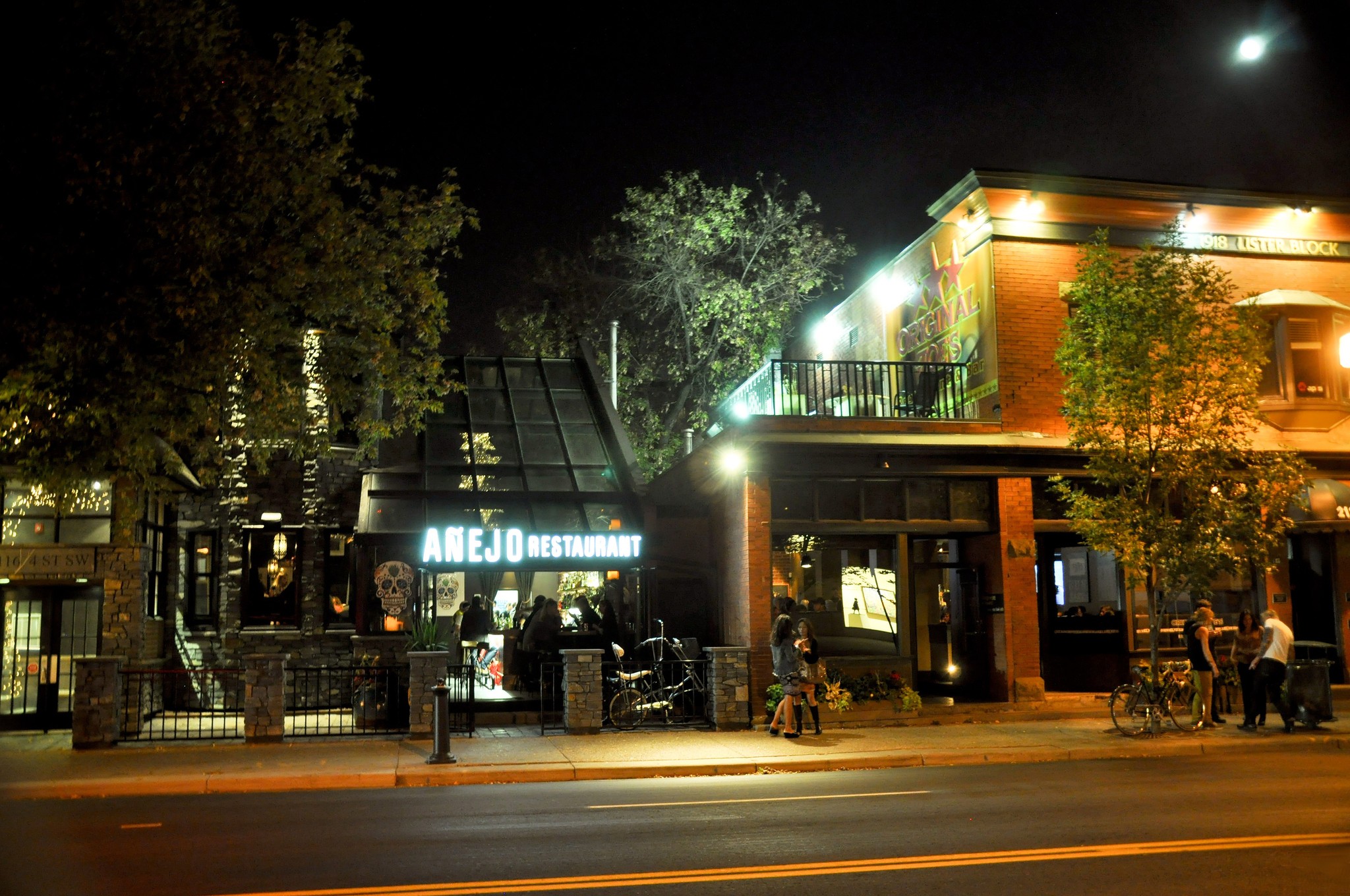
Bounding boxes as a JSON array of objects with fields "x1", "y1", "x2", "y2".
[{"x1": 801, "y1": 553, "x2": 812, "y2": 568}]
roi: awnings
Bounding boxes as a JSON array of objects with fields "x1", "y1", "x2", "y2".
[{"x1": 1284, "y1": 478, "x2": 1350, "y2": 534}]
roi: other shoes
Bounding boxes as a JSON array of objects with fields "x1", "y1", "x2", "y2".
[
  {"x1": 1203, "y1": 722, "x2": 1223, "y2": 729},
  {"x1": 1237, "y1": 723, "x2": 1256, "y2": 731},
  {"x1": 1242, "y1": 715, "x2": 1246, "y2": 720},
  {"x1": 475, "y1": 668, "x2": 490, "y2": 675},
  {"x1": 1191, "y1": 721, "x2": 1203, "y2": 727},
  {"x1": 1258, "y1": 721, "x2": 1265, "y2": 725},
  {"x1": 1282, "y1": 721, "x2": 1293, "y2": 732},
  {"x1": 1212, "y1": 716, "x2": 1226, "y2": 723}
]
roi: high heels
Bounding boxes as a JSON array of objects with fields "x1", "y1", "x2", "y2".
[
  {"x1": 784, "y1": 732, "x2": 800, "y2": 738},
  {"x1": 769, "y1": 727, "x2": 779, "y2": 735}
]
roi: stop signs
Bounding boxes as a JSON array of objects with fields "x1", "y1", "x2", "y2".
[{"x1": 28, "y1": 664, "x2": 38, "y2": 674}]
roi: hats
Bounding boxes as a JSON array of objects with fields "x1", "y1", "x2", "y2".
[
  {"x1": 784, "y1": 597, "x2": 796, "y2": 611},
  {"x1": 811, "y1": 596, "x2": 825, "y2": 604}
]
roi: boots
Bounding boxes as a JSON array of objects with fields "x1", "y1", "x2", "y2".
[
  {"x1": 809, "y1": 705, "x2": 822, "y2": 734},
  {"x1": 792, "y1": 704, "x2": 802, "y2": 734}
]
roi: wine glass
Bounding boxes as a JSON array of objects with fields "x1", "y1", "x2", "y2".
[{"x1": 578, "y1": 616, "x2": 584, "y2": 631}]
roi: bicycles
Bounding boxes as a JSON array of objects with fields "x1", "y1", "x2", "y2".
[
  {"x1": 1107, "y1": 659, "x2": 1206, "y2": 736},
  {"x1": 603, "y1": 618, "x2": 707, "y2": 731}
]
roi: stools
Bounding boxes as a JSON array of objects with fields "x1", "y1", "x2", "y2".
[{"x1": 462, "y1": 640, "x2": 495, "y2": 690}]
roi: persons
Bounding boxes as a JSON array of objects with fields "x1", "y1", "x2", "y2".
[
  {"x1": 1230, "y1": 609, "x2": 1266, "y2": 725},
  {"x1": 940, "y1": 591, "x2": 951, "y2": 623},
  {"x1": 793, "y1": 618, "x2": 822, "y2": 735},
  {"x1": 1182, "y1": 599, "x2": 1226, "y2": 730},
  {"x1": 1058, "y1": 604, "x2": 1115, "y2": 616},
  {"x1": 522, "y1": 594, "x2": 562, "y2": 648},
  {"x1": 592, "y1": 599, "x2": 620, "y2": 644},
  {"x1": 453, "y1": 596, "x2": 489, "y2": 649},
  {"x1": 769, "y1": 614, "x2": 805, "y2": 738},
  {"x1": 773, "y1": 596, "x2": 837, "y2": 614},
  {"x1": 572, "y1": 596, "x2": 602, "y2": 629},
  {"x1": 1237, "y1": 610, "x2": 1295, "y2": 733}
]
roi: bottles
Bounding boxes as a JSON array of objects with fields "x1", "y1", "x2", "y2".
[
  {"x1": 558, "y1": 576, "x2": 584, "y2": 609},
  {"x1": 494, "y1": 613, "x2": 512, "y2": 629}
]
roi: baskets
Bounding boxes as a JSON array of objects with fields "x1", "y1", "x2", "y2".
[{"x1": 677, "y1": 638, "x2": 699, "y2": 660}]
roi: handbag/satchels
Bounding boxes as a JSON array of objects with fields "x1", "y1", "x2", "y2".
[
  {"x1": 852, "y1": 606, "x2": 855, "y2": 609},
  {"x1": 797, "y1": 657, "x2": 827, "y2": 683}
]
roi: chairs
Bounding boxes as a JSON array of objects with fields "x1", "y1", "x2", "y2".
[{"x1": 893, "y1": 371, "x2": 940, "y2": 418}]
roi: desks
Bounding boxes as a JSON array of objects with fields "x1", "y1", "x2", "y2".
[
  {"x1": 826, "y1": 394, "x2": 891, "y2": 417},
  {"x1": 557, "y1": 630, "x2": 597, "y2": 648}
]
